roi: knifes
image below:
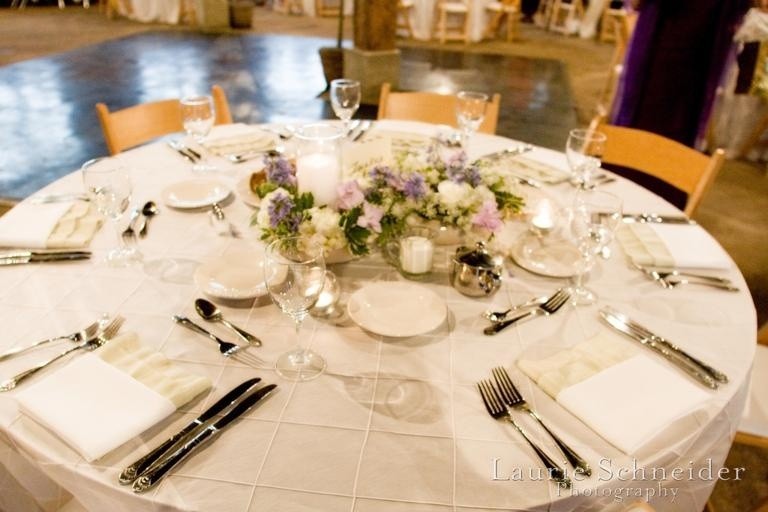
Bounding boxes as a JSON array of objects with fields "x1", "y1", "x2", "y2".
[
  {"x1": 2, "y1": 251, "x2": 92, "y2": 268},
  {"x1": 593, "y1": 302, "x2": 731, "y2": 390},
  {"x1": 166, "y1": 138, "x2": 203, "y2": 168},
  {"x1": 348, "y1": 117, "x2": 373, "y2": 142},
  {"x1": 118, "y1": 375, "x2": 263, "y2": 484},
  {"x1": 133, "y1": 383, "x2": 278, "y2": 494},
  {"x1": 596, "y1": 205, "x2": 692, "y2": 225}
]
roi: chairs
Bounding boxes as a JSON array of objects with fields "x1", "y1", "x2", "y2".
[
  {"x1": 372, "y1": 80, "x2": 500, "y2": 133},
  {"x1": 31, "y1": 1, "x2": 767, "y2": 110},
  {"x1": 582, "y1": 104, "x2": 724, "y2": 217},
  {"x1": 95, "y1": 85, "x2": 231, "y2": 153}
]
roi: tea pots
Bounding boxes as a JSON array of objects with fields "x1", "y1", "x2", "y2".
[{"x1": 451, "y1": 240, "x2": 503, "y2": 296}]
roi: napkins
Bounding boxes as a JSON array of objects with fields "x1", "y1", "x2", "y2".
[{"x1": 0, "y1": 121, "x2": 759, "y2": 511}]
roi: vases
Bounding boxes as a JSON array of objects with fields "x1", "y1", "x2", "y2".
[
  {"x1": 404, "y1": 217, "x2": 484, "y2": 247},
  {"x1": 280, "y1": 236, "x2": 373, "y2": 267}
]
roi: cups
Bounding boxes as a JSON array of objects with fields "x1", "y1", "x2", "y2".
[
  {"x1": 81, "y1": 157, "x2": 142, "y2": 267},
  {"x1": 295, "y1": 124, "x2": 344, "y2": 205},
  {"x1": 182, "y1": 97, "x2": 217, "y2": 172},
  {"x1": 397, "y1": 224, "x2": 440, "y2": 276},
  {"x1": 564, "y1": 129, "x2": 606, "y2": 188}
]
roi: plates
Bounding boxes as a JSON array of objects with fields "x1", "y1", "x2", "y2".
[
  {"x1": 343, "y1": 280, "x2": 449, "y2": 337},
  {"x1": 242, "y1": 159, "x2": 387, "y2": 266},
  {"x1": 2, "y1": 95, "x2": 389, "y2": 317},
  {"x1": 509, "y1": 235, "x2": 592, "y2": 278},
  {"x1": 161, "y1": 179, "x2": 230, "y2": 211},
  {"x1": 195, "y1": 246, "x2": 273, "y2": 301}
]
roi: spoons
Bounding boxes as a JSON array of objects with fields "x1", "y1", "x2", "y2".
[
  {"x1": 484, "y1": 296, "x2": 549, "y2": 324},
  {"x1": 194, "y1": 296, "x2": 262, "y2": 348}
]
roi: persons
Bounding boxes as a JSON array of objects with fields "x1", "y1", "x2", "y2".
[
  {"x1": 601, "y1": 0, "x2": 749, "y2": 212},
  {"x1": 606, "y1": 0, "x2": 627, "y2": 16},
  {"x1": 517, "y1": 0, "x2": 539, "y2": 25}
]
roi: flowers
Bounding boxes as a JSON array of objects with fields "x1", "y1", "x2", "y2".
[
  {"x1": 246, "y1": 144, "x2": 387, "y2": 259},
  {"x1": 355, "y1": 135, "x2": 529, "y2": 251}
]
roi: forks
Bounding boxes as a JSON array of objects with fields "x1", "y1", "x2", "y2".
[
  {"x1": 631, "y1": 265, "x2": 743, "y2": 295},
  {"x1": 0, "y1": 321, "x2": 110, "y2": 364},
  {"x1": 475, "y1": 379, "x2": 571, "y2": 490},
  {"x1": 482, "y1": 288, "x2": 571, "y2": 337},
  {"x1": 490, "y1": 364, "x2": 592, "y2": 478},
  {"x1": 0, "y1": 315, "x2": 128, "y2": 394},
  {"x1": 169, "y1": 315, "x2": 266, "y2": 372}
]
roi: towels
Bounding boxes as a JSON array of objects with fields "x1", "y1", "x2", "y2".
[
  {"x1": 9, "y1": 327, "x2": 213, "y2": 463},
  {"x1": 507, "y1": 141, "x2": 602, "y2": 188},
  {"x1": 200, "y1": 118, "x2": 277, "y2": 159},
  {"x1": 613, "y1": 218, "x2": 732, "y2": 278},
  {"x1": 513, "y1": 324, "x2": 715, "y2": 460},
  {"x1": 0, "y1": 187, "x2": 131, "y2": 252}
]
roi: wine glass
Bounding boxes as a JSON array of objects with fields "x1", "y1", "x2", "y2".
[
  {"x1": 322, "y1": 369, "x2": 433, "y2": 423},
  {"x1": 329, "y1": 76, "x2": 363, "y2": 140},
  {"x1": 565, "y1": 188, "x2": 625, "y2": 308},
  {"x1": 260, "y1": 233, "x2": 328, "y2": 384},
  {"x1": 455, "y1": 89, "x2": 489, "y2": 151}
]
